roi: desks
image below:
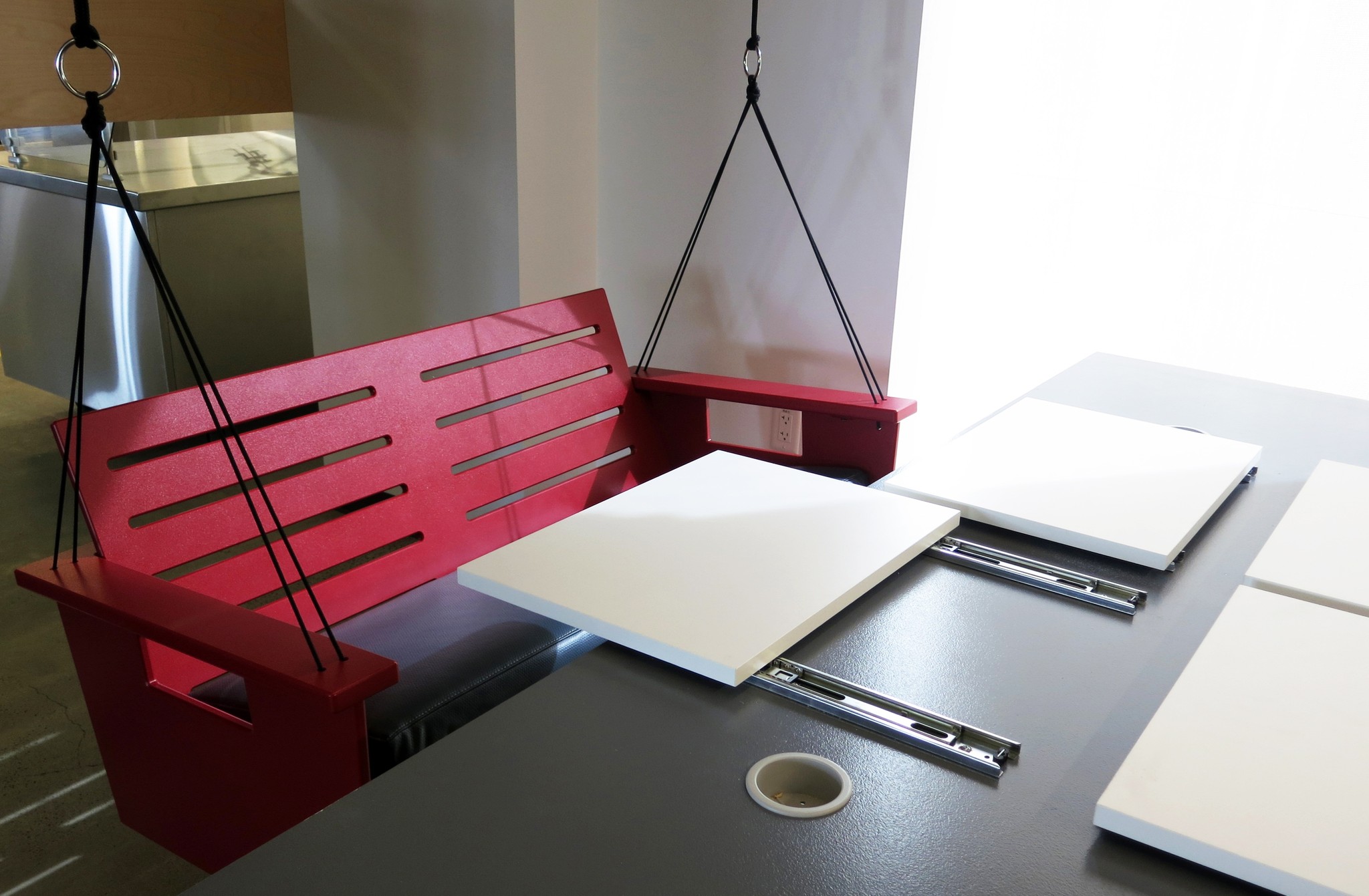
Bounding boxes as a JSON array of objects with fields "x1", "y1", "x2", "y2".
[{"x1": 184, "y1": 342, "x2": 1369, "y2": 896}]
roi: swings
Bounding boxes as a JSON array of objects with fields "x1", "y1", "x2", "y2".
[{"x1": 12, "y1": 1, "x2": 918, "y2": 875}]
